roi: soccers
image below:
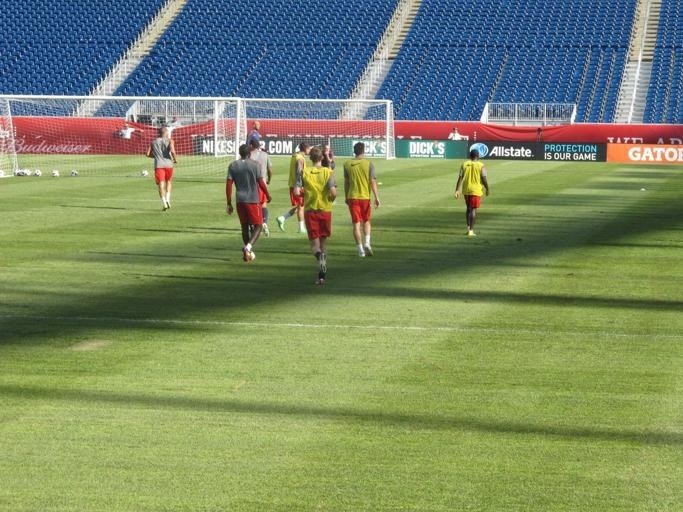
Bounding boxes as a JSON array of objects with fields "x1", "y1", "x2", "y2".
[
  {"x1": 71, "y1": 170, "x2": 78, "y2": 176},
  {"x1": 24, "y1": 169, "x2": 31, "y2": 176},
  {"x1": 13, "y1": 168, "x2": 20, "y2": 175},
  {"x1": 18, "y1": 171, "x2": 24, "y2": 175},
  {"x1": 34, "y1": 169, "x2": 41, "y2": 176},
  {"x1": 52, "y1": 171, "x2": 59, "y2": 177},
  {"x1": 141, "y1": 168, "x2": 148, "y2": 176}
]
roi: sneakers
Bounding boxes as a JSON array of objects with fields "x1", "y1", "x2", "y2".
[
  {"x1": 162, "y1": 201, "x2": 171, "y2": 211},
  {"x1": 241, "y1": 246, "x2": 256, "y2": 262},
  {"x1": 276, "y1": 216, "x2": 285, "y2": 232},
  {"x1": 465, "y1": 226, "x2": 477, "y2": 237},
  {"x1": 297, "y1": 228, "x2": 308, "y2": 234},
  {"x1": 358, "y1": 250, "x2": 365, "y2": 258},
  {"x1": 261, "y1": 222, "x2": 269, "y2": 237},
  {"x1": 318, "y1": 252, "x2": 328, "y2": 275},
  {"x1": 363, "y1": 243, "x2": 373, "y2": 256}
]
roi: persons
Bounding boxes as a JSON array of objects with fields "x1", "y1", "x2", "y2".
[
  {"x1": 246, "y1": 121, "x2": 261, "y2": 144},
  {"x1": 170, "y1": 113, "x2": 177, "y2": 125},
  {"x1": 119, "y1": 123, "x2": 142, "y2": 140},
  {"x1": 491, "y1": 103, "x2": 573, "y2": 118},
  {"x1": 248, "y1": 139, "x2": 271, "y2": 237},
  {"x1": 321, "y1": 143, "x2": 335, "y2": 168},
  {"x1": 455, "y1": 150, "x2": 489, "y2": 235},
  {"x1": 447, "y1": 127, "x2": 469, "y2": 141},
  {"x1": 537, "y1": 128, "x2": 542, "y2": 144},
  {"x1": 276, "y1": 142, "x2": 311, "y2": 235},
  {"x1": 293, "y1": 147, "x2": 337, "y2": 287},
  {"x1": 225, "y1": 145, "x2": 271, "y2": 262},
  {"x1": 342, "y1": 142, "x2": 379, "y2": 258},
  {"x1": 145, "y1": 127, "x2": 176, "y2": 211}
]
